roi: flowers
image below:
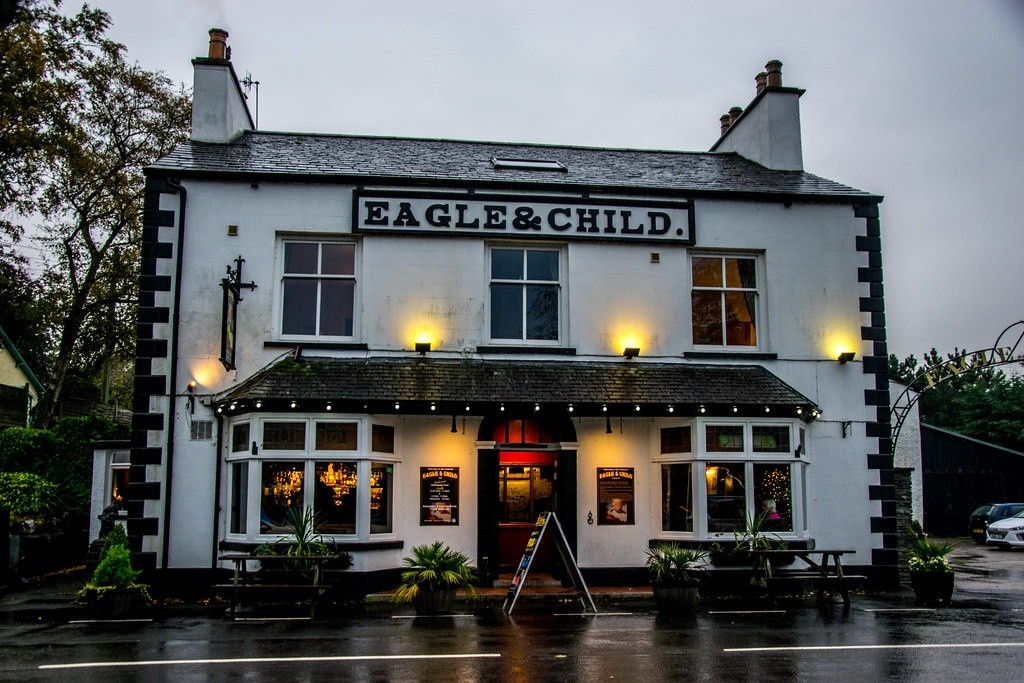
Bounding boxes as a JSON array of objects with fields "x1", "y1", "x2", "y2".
[{"x1": 907, "y1": 555, "x2": 950, "y2": 572}]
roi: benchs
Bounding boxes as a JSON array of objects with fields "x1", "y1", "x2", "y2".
[
  {"x1": 215, "y1": 578, "x2": 331, "y2": 590},
  {"x1": 761, "y1": 571, "x2": 867, "y2": 581}
]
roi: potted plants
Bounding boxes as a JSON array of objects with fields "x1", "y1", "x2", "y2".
[
  {"x1": 896, "y1": 520, "x2": 959, "y2": 605},
  {"x1": 732, "y1": 503, "x2": 785, "y2": 605},
  {"x1": 391, "y1": 542, "x2": 478, "y2": 615},
  {"x1": 642, "y1": 541, "x2": 708, "y2": 611},
  {"x1": 77, "y1": 524, "x2": 155, "y2": 615}
]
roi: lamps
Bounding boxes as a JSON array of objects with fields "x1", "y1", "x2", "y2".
[
  {"x1": 415, "y1": 342, "x2": 431, "y2": 355},
  {"x1": 624, "y1": 348, "x2": 640, "y2": 359},
  {"x1": 838, "y1": 352, "x2": 856, "y2": 364}
]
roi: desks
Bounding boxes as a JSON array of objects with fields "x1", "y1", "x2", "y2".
[
  {"x1": 218, "y1": 554, "x2": 341, "y2": 623},
  {"x1": 747, "y1": 549, "x2": 857, "y2": 607}
]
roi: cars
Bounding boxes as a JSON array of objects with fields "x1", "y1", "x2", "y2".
[
  {"x1": 968, "y1": 503, "x2": 1024, "y2": 545},
  {"x1": 986, "y1": 509, "x2": 1024, "y2": 550}
]
